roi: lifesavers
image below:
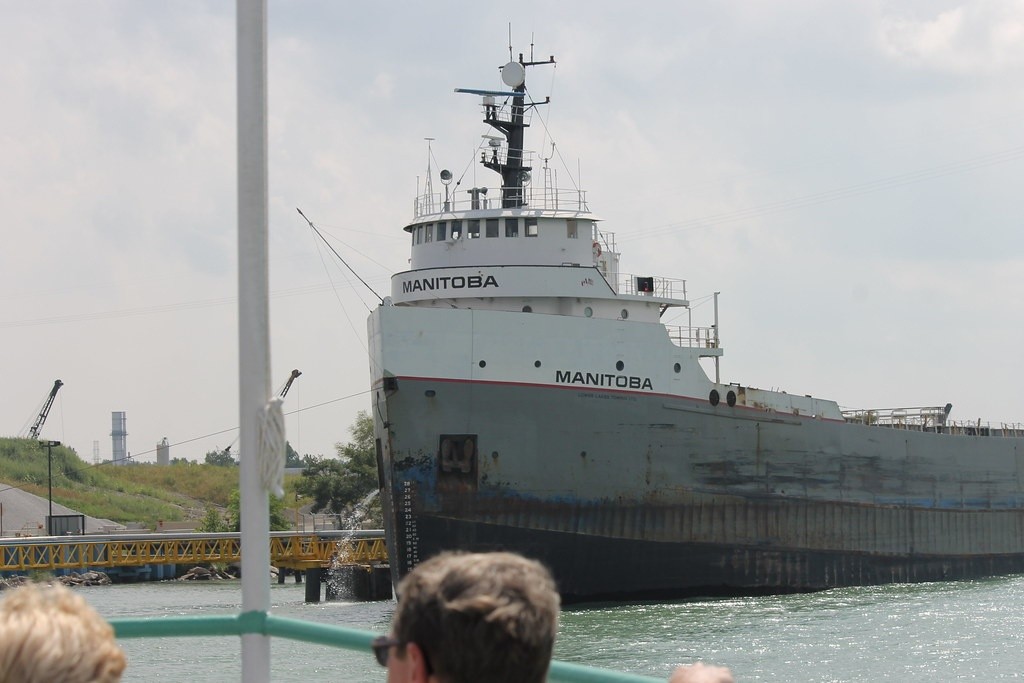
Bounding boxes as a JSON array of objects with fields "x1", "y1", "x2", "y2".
[{"x1": 593, "y1": 243, "x2": 601, "y2": 257}]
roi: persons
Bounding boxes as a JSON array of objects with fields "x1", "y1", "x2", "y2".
[
  {"x1": 372, "y1": 549, "x2": 734, "y2": 683},
  {"x1": 0, "y1": 577, "x2": 128, "y2": 683}
]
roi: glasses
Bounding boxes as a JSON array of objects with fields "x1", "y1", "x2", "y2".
[{"x1": 372, "y1": 636, "x2": 397, "y2": 667}]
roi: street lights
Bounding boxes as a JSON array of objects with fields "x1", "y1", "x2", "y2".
[
  {"x1": 295, "y1": 494, "x2": 299, "y2": 535},
  {"x1": 38, "y1": 441, "x2": 61, "y2": 536}
]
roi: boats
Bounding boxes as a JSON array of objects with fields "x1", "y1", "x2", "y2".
[{"x1": 366, "y1": 22, "x2": 1023, "y2": 610}]
